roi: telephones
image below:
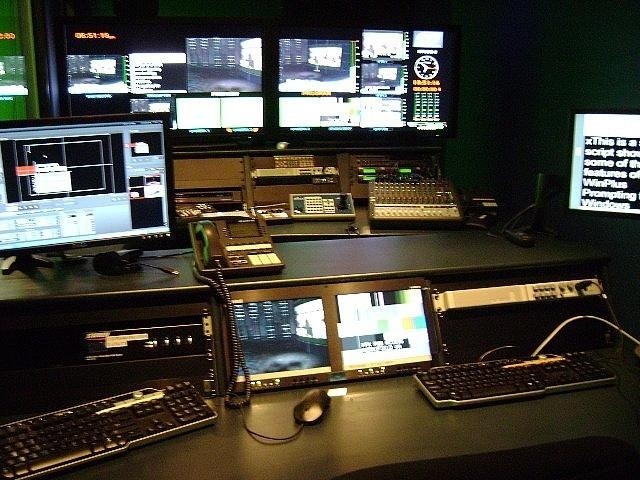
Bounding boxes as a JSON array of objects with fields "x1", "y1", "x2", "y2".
[{"x1": 188, "y1": 217, "x2": 285, "y2": 280}]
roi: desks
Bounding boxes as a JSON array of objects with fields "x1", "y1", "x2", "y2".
[
  {"x1": 48, "y1": 350, "x2": 640, "y2": 480},
  {"x1": 268, "y1": 203, "x2": 505, "y2": 236},
  {"x1": 1, "y1": 233, "x2": 638, "y2": 299}
]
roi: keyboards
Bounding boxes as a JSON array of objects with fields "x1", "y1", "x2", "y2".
[
  {"x1": 0, "y1": 381, "x2": 219, "y2": 480},
  {"x1": 414, "y1": 352, "x2": 619, "y2": 410}
]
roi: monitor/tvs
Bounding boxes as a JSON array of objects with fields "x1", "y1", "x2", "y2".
[
  {"x1": 565, "y1": 110, "x2": 640, "y2": 220},
  {"x1": 64, "y1": 31, "x2": 445, "y2": 136},
  {"x1": 232, "y1": 297, "x2": 331, "y2": 383},
  {"x1": 335, "y1": 286, "x2": 434, "y2": 370},
  {"x1": 0, "y1": 112, "x2": 176, "y2": 275}
]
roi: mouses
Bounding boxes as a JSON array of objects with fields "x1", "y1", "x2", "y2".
[{"x1": 294, "y1": 389, "x2": 330, "y2": 425}]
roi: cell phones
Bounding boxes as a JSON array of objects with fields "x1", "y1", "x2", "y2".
[{"x1": 505, "y1": 229, "x2": 536, "y2": 247}]
